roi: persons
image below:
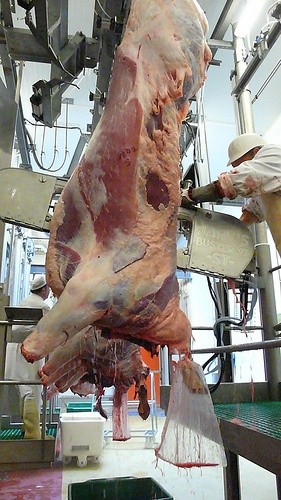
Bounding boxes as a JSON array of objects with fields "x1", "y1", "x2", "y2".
[{"x1": 181, "y1": 132, "x2": 281, "y2": 259}]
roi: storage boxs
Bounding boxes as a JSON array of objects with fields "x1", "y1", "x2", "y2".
[{"x1": 59, "y1": 402, "x2": 106, "y2": 467}]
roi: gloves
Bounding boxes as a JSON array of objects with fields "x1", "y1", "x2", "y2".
[{"x1": 177, "y1": 181, "x2": 218, "y2": 206}]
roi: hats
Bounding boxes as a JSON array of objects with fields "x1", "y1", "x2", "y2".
[
  {"x1": 227, "y1": 132, "x2": 267, "y2": 167},
  {"x1": 30, "y1": 275, "x2": 46, "y2": 291}
]
